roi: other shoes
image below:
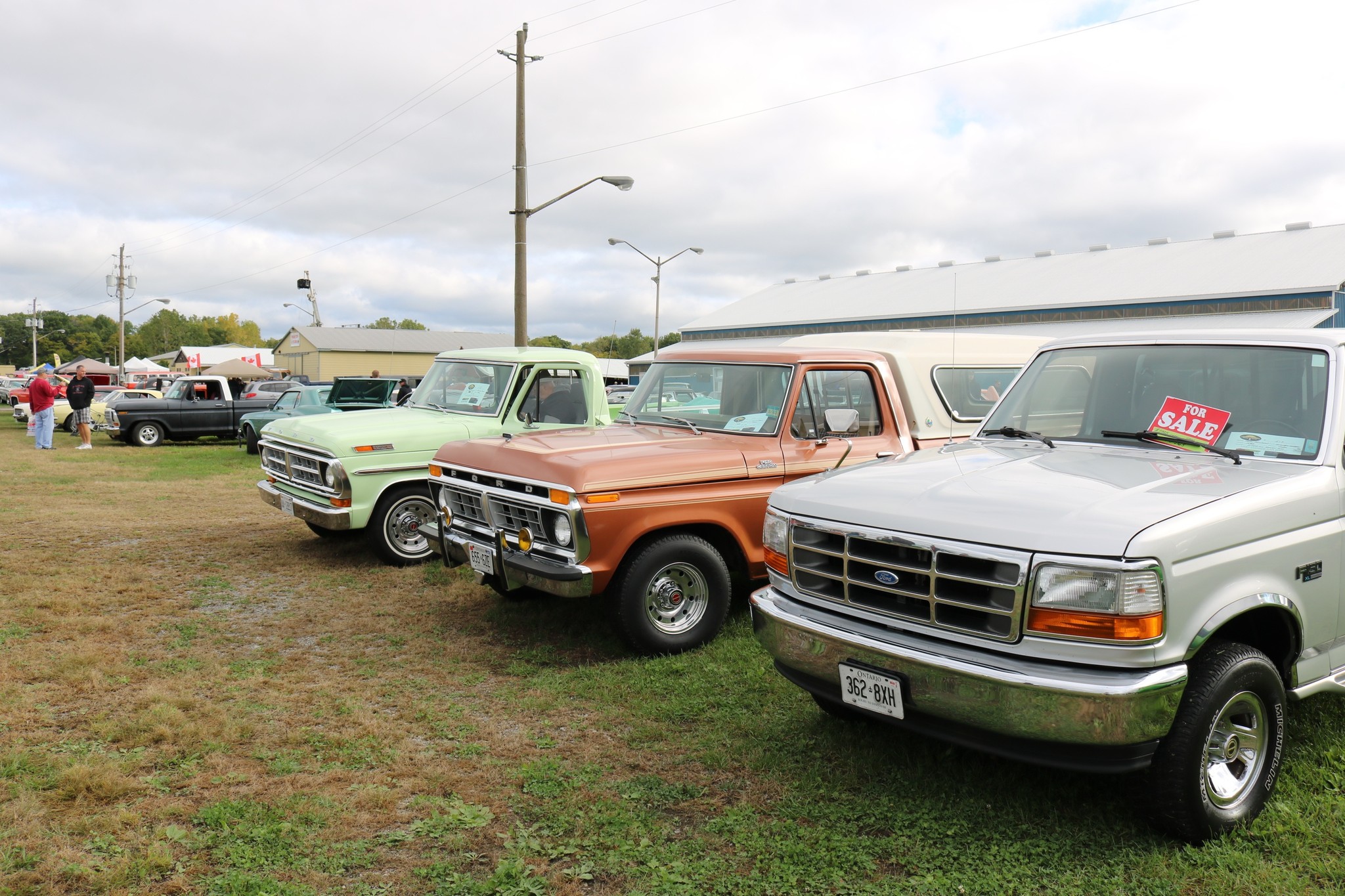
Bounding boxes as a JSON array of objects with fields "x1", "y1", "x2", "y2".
[
  {"x1": 75, "y1": 443, "x2": 92, "y2": 449},
  {"x1": 42, "y1": 446, "x2": 56, "y2": 450}
]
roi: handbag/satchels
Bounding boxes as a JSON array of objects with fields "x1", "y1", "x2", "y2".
[{"x1": 26, "y1": 415, "x2": 36, "y2": 437}]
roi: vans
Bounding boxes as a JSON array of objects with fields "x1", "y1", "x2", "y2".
[{"x1": 124, "y1": 371, "x2": 187, "y2": 389}]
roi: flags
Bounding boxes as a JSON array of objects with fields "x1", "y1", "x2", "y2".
[
  {"x1": 187, "y1": 353, "x2": 201, "y2": 369},
  {"x1": 241, "y1": 353, "x2": 262, "y2": 368}
]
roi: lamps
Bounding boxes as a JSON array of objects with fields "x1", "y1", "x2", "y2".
[{"x1": 297, "y1": 279, "x2": 311, "y2": 288}]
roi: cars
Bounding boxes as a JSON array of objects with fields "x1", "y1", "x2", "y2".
[
  {"x1": 746, "y1": 328, "x2": 1345, "y2": 846},
  {"x1": 0, "y1": 372, "x2": 207, "y2": 433},
  {"x1": 604, "y1": 382, "x2": 721, "y2": 403},
  {"x1": 235, "y1": 369, "x2": 417, "y2": 455}
]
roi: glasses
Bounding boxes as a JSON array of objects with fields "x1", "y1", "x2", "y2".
[{"x1": 532, "y1": 383, "x2": 545, "y2": 392}]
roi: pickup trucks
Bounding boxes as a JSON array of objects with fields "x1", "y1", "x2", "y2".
[
  {"x1": 256, "y1": 346, "x2": 720, "y2": 568},
  {"x1": 104, "y1": 375, "x2": 277, "y2": 447},
  {"x1": 274, "y1": 375, "x2": 334, "y2": 388},
  {"x1": 417, "y1": 332, "x2": 1094, "y2": 655}
]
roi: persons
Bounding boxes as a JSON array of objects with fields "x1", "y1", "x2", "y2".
[
  {"x1": 530, "y1": 370, "x2": 572, "y2": 423},
  {"x1": 621, "y1": 383, "x2": 623, "y2": 385},
  {"x1": 613, "y1": 382, "x2": 618, "y2": 385},
  {"x1": 993, "y1": 380, "x2": 1003, "y2": 398},
  {"x1": 372, "y1": 369, "x2": 380, "y2": 378},
  {"x1": 66, "y1": 365, "x2": 95, "y2": 449},
  {"x1": 396, "y1": 378, "x2": 412, "y2": 406},
  {"x1": 29, "y1": 367, "x2": 66, "y2": 450},
  {"x1": 226, "y1": 377, "x2": 247, "y2": 401},
  {"x1": 968, "y1": 370, "x2": 981, "y2": 401}
]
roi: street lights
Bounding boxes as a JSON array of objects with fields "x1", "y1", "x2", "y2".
[
  {"x1": 515, "y1": 177, "x2": 634, "y2": 380},
  {"x1": 284, "y1": 303, "x2": 315, "y2": 327},
  {"x1": 33, "y1": 330, "x2": 65, "y2": 369},
  {"x1": 607, "y1": 238, "x2": 703, "y2": 388},
  {"x1": 118, "y1": 299, "x2": 171, "y2": 386}
]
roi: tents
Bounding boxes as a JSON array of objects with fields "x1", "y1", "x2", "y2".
[
  {"x1": 28, "y1": 355, "x2": 120, "y2": 386},
  {"x1": 201, "y1": 358, "x2": 274, "y2": 383},
  {"x1": 141, "y1": 357, "x2": 171, "y2": 372},
  {"x1": 108, "y1": 356, "x2": 147, "y2": 387}
]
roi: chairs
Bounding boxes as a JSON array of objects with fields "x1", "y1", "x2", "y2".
[
  {"x1": 511, "y1": 378, "x2": 541, "y2": 418},
  {"x1": 818, "y1": 409, "x2": 860, "y2": 439},
  {"x1": 533, "y1": 381, "x2": 588, "y2": 424}
]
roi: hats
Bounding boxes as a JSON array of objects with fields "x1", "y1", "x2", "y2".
[
  {"x1": 38, "y1": 367, "x2": 50, "y2": 373},
  {"x1": 398, "y1": 379, "x2": 406, "y2": 383}
]
